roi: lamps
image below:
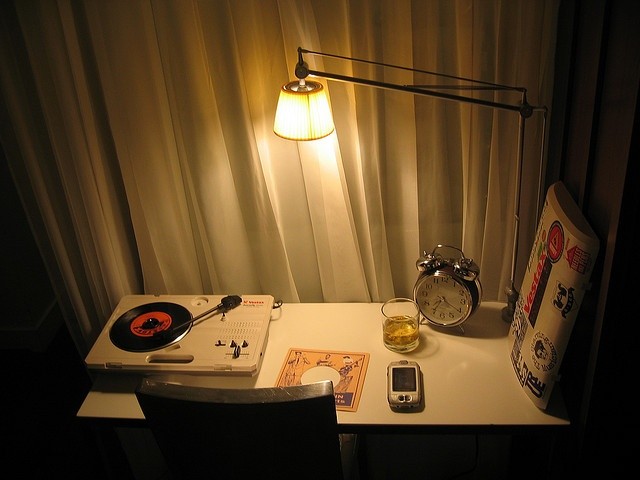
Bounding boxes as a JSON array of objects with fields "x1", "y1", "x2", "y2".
[{"x1": 132, "y1": 377, "x2": 355, "y2": 480}]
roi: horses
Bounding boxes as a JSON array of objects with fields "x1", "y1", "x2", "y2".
[{"x1": 387, "y1": 360, "x2": 421, "y2": 408}]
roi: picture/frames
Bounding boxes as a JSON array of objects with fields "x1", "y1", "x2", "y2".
[{"x1": 75, "y1": 302, "x2": 575, "y2": 429}]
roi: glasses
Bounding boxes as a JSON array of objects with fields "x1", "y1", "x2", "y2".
[{"x1": 270, "y1": 44, "x2": 548, "y2": 320}]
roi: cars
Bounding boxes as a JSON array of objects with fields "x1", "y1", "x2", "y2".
[{"x1": 381, "y1": 298, "x2": 420, "y2": 354}]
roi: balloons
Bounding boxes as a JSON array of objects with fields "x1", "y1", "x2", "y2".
[{"x1": 412, "y1": 245, "x2": 484, "y2": 332}]
